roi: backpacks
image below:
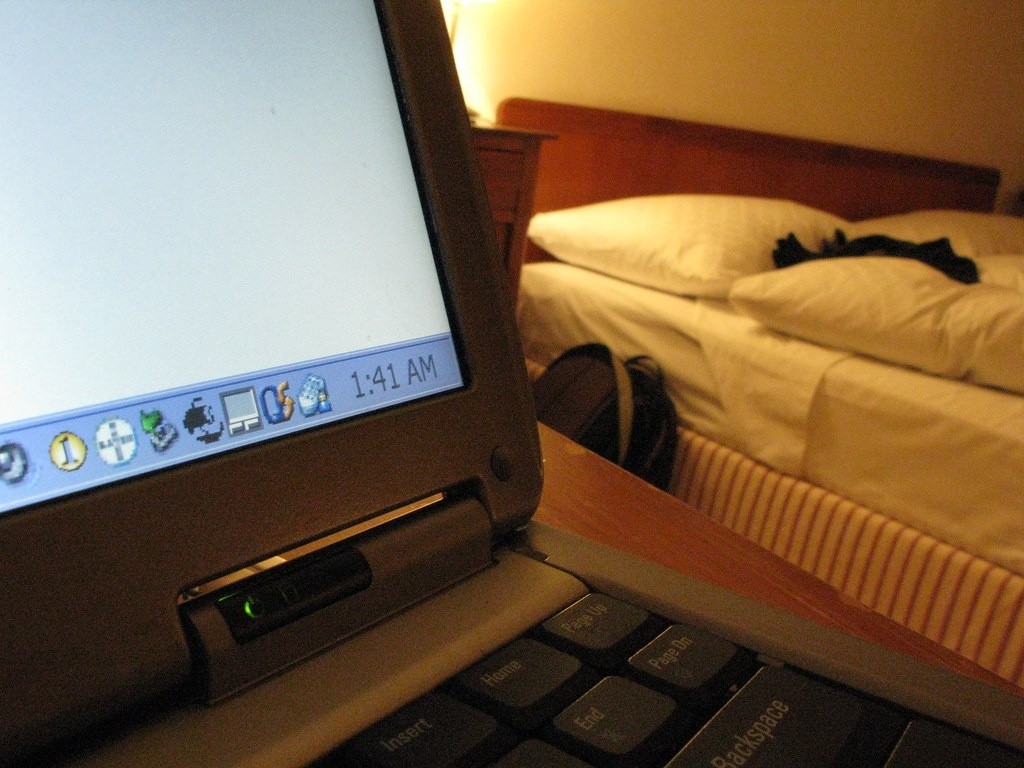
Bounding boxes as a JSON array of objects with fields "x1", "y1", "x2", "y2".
[{"x1": 528, "y1": 343, "x2": 676, "y2": 491}]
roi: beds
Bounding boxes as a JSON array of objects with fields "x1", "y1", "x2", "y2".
[{"x1": 497, "y1": 98, "x2": 1024, "y2": 689}]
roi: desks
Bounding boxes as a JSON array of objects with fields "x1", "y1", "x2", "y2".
[{"x1": 531, "y1": 420, "x2": 1024, "y2": 697}]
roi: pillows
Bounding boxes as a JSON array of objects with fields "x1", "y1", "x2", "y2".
[
  {"x1": 527, "y1": 192, "x2": 855, "y2": 303},
  {"x1": 858, "y1": 210, "x2": 1024, "y2": 258},
  {"x1": 726, "y1": 257, "x2": 1024, "y2": 394}
]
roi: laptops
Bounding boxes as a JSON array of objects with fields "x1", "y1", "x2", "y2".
[{"x1": 0, "y1": 0, "x2": 1024, "y2": 768}]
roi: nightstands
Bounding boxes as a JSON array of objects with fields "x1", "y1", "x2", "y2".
[{"x1": 473, "y1": 121, "x2": 560, "y2": 308}]
